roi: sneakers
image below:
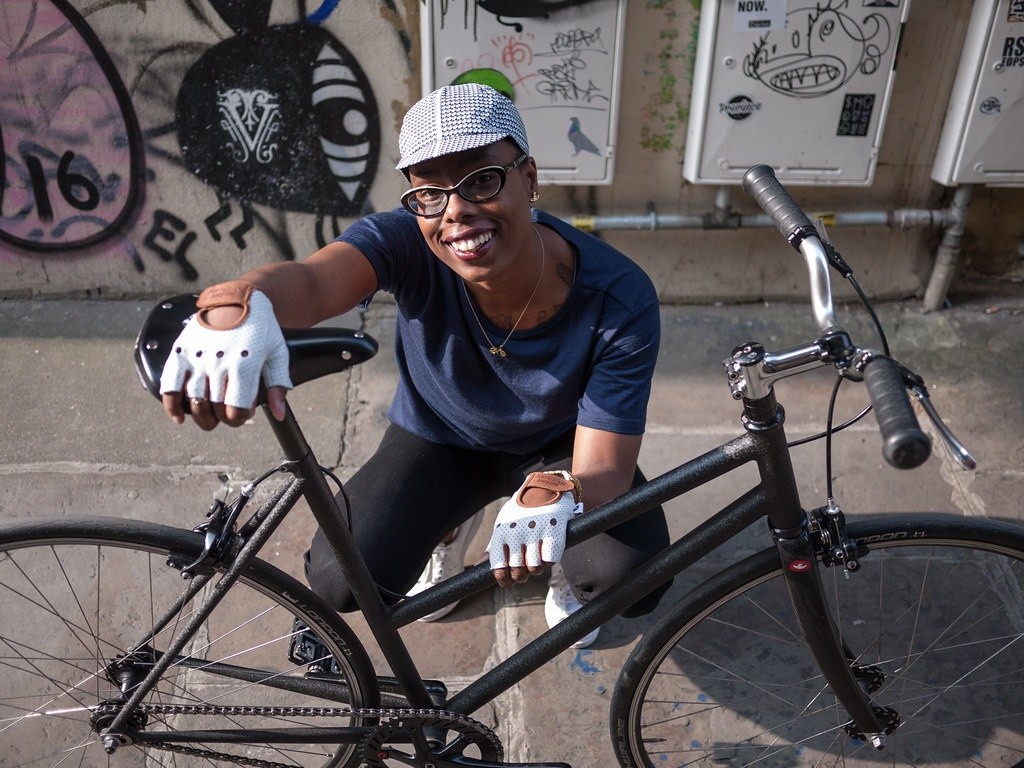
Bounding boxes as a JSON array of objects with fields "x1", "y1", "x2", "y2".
[
  {"x1": 545, "y1": 560, "x2": 601, "y2": 648},
  {"x1": 402, "y1": 507, "x2": 484, "y2": 624}
]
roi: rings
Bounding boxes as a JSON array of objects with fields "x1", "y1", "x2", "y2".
[{"x1": 189, "y1": 396, "x2": 208, "y2": 405}]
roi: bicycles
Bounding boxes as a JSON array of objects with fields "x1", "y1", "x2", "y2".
[{"x1": 0, "y1": 162, "x2": 1024, "y2": 768}]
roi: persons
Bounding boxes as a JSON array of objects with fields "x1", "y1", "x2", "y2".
[{"x1": 160, "y1": 85, "x2": 669, "y2": 649}]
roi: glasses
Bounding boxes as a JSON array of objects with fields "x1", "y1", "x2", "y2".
[{"x1": 400, "y1": 153, "x2": 529, "y2": 219}]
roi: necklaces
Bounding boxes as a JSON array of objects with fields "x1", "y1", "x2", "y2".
[{"x1": 463, "y1": 223, "x2": 545, "y2": 357}]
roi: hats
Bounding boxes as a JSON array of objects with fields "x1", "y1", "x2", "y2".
[{"x1": 398, "y1": 82, "x2": 529, "y2": 185}]
